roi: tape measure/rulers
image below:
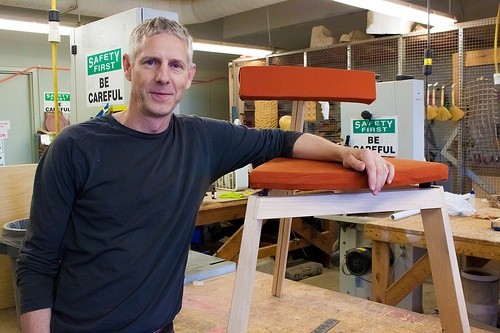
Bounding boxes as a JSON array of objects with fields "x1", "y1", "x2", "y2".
[{"x1": 311, "y1": 318, "x2": 340, "y2": 333}]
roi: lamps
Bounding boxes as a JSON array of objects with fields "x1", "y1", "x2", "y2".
[
  {"x1": 192, "y1": 7, "x2": 274, "y2": 57},
  {"x1": 333, "y1": 0, "x2": 458, "y2": 27}
]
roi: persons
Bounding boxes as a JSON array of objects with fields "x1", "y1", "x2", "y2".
[{"x1": 15, "y1": 17, "x2": 395, "y2": 333}]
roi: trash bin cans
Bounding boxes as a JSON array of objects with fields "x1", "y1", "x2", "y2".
[{"x1": 0, "y1": 217, "x2": 29, "y2": 330}]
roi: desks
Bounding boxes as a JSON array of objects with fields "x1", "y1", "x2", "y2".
[
  {"x1": 196, "y1": 190, "x2": 339, "y2": 269},
  {"x1": 173, "y1": 270, "x2": 495, "y2": 333},
  {"x1": 363, "y1": 197, "x2": 500, "y2": 307}
]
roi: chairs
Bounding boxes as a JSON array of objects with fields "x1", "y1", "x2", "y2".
[{"x1": 226, "y1": 66, "x2": 472, "y2": 333}]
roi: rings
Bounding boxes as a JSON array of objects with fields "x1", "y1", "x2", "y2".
[{"x1": 381, "y1": 164, "x2": 388, "y2": 168}]
